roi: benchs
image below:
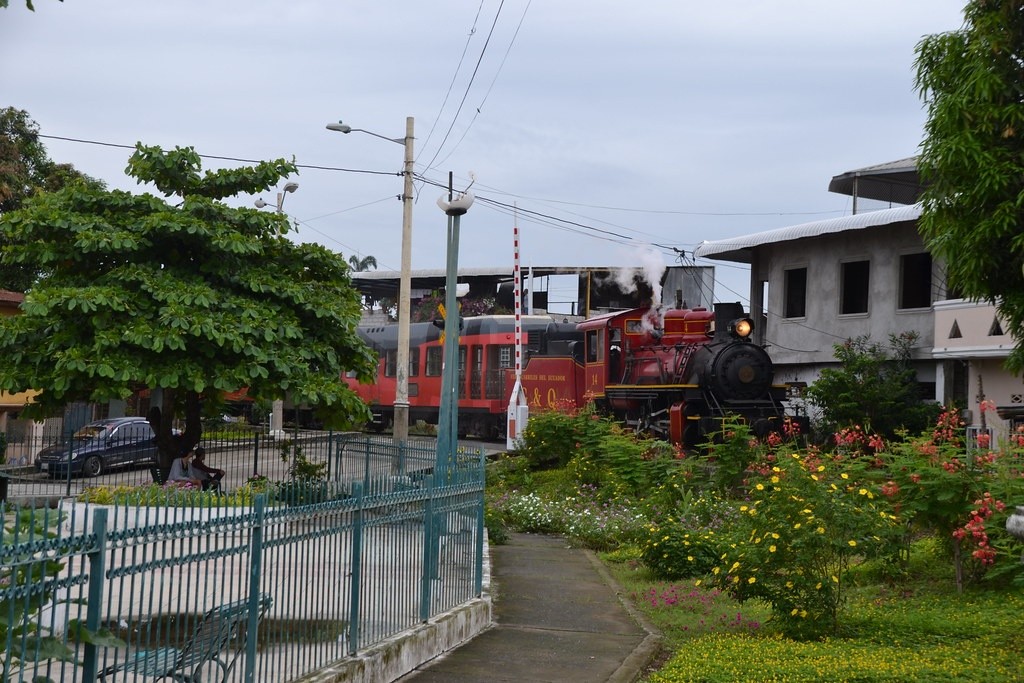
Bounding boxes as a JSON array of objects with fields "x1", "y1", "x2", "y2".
[
  {"x1": 149, "y1": 462, "x2": 204, "y2": 486},
  {"x1": 97, "y1": 591, "x2": 273, "y2": 683}
]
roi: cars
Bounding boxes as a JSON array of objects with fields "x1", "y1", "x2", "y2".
[{"x1": 35, "y1": 415, "x2": 182, "y2": 477}]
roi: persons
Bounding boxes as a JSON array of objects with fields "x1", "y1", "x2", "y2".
[{"x1": 168, "y1": 446, "x2": 221, "y2": 492}]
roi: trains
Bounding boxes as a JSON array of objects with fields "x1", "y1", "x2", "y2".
[{"x1": 217, "y1": 260, "x2": 811, "y2": 448}]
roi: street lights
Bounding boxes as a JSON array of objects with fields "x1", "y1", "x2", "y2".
[
  {"x1": 254, "y1": 181, "x2": 299, "y2": 431},
  {"x1": 141, "y1": 121, "x2": 416, "y2": 473}
]
